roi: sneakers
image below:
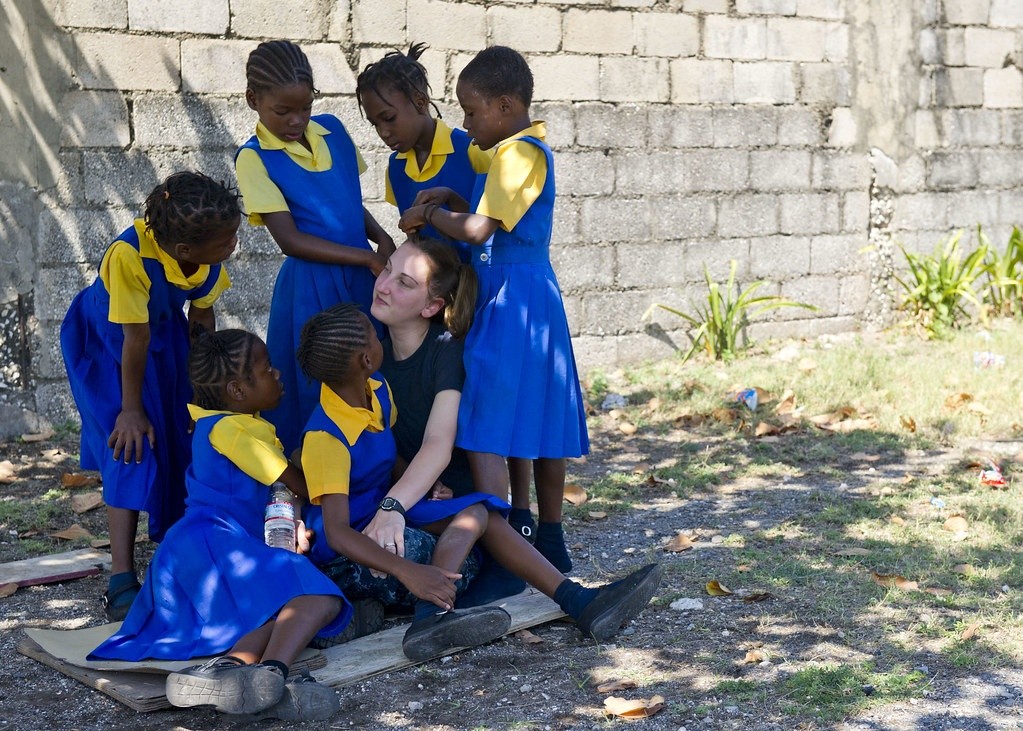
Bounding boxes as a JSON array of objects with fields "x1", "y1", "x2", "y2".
[
  {"x1": 402, "y1": 606, "x2": 512, "y2": 661},
  {"x1": 578, "y1": 567, "x2": 660, "y2": 639}
]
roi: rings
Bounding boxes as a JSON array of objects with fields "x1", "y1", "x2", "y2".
[{"x1": 385, "y1": 543, "x2": 395, "y2": 547}]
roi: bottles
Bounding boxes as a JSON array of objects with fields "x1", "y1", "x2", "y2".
[{"x1": 265, "y1": 482, "x2": 295, "y2": 554}]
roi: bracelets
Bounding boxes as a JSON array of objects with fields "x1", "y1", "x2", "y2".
[{"x1": 423, "y1": 203, "x2": 440, "y2": 224}]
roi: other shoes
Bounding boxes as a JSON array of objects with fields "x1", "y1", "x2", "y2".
[
  {"x1": 312, "y1": 598, "x2": 385, "y2": 649},
  {"x1": 536, "y1": 524, "x2": 572, "y2": 572},
  {"x1": 509, "y1": 518, "x2": 536, "y2": 545},
  {"x1": 103, "y1": 583, "x2": 144, "y2": 619},
  {"x1": 234, "y1": 676, "x2": 340, "y2": 724},
  {"x1": 166, "y1": 654, "x2": 285, "y2": 715}
]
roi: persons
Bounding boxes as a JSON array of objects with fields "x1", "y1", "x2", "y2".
[
  {"x1": 357, "y1": 43, "x2": 476, "y2": 258},
  {"x1": 307, "y1": 230, "x2": 485, "y2": 648},
  {"x1": 297, "y1": 308, "x2": 665, "y2": 662},
  {"x1": 398, "y1": 46, "x2": 590, "y2": 574},
  {"x1": 87, "y1": 329, "x2": 358, "y2": 712},
  {"x1": 235, "y1": 40, "x2": 395, "y2": 451},
  {"x1": 60, "y1": 170, "x2": 250, "y2": 621}
]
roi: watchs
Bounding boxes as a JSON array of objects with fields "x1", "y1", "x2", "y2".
[{"x1": 377, "y1": 497, "x2": 406, "y2": 518}]
